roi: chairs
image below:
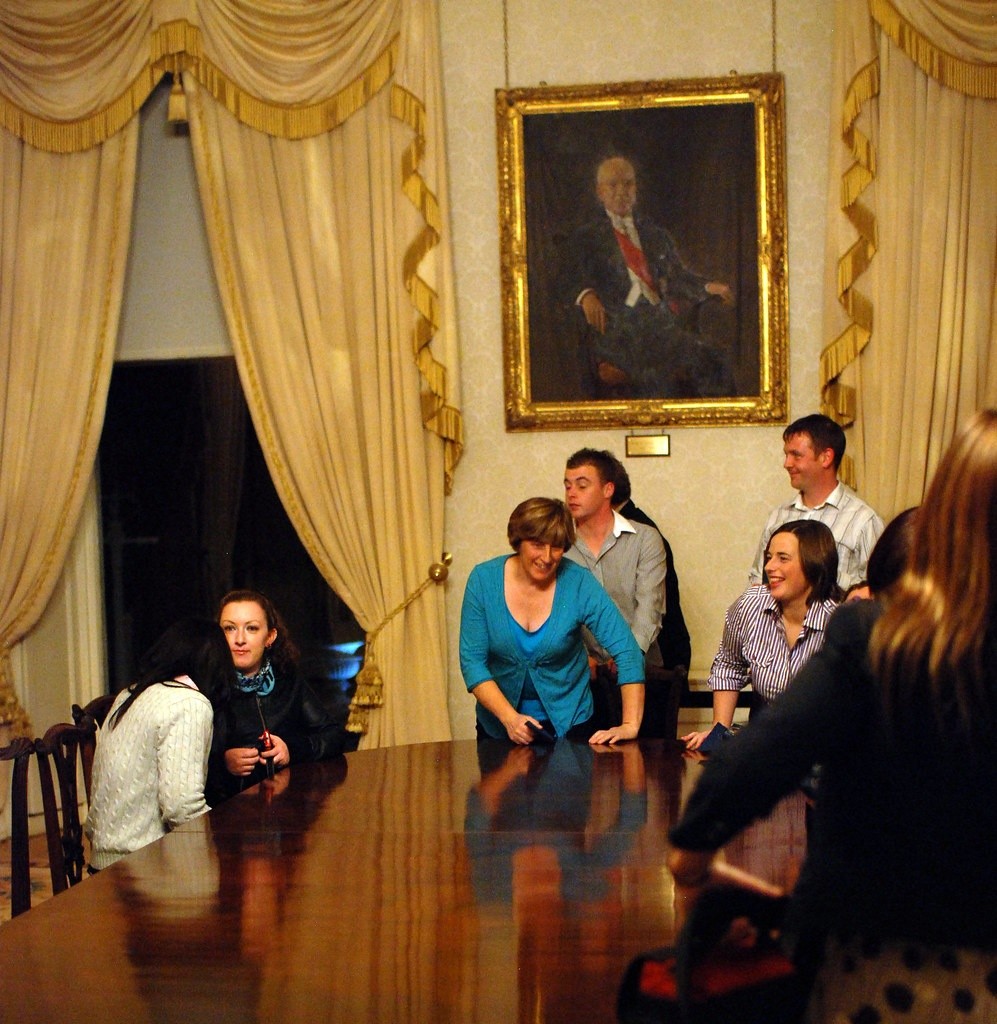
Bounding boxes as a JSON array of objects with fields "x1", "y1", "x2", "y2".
[
  {"x1": 71, "y1": 695, "x2": 117, "y2": 807},
  {"x1": 0, "y1": 723, "x2": 89, "y2": 919}
]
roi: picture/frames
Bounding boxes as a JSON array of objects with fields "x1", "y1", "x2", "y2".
[{"x1": 493, "y1": 72, "x2": 790, "y2": 436}]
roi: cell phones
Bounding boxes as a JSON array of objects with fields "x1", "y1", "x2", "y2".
[{"x1": 525, "y1": 721, "x2": 553, "y2": 741}]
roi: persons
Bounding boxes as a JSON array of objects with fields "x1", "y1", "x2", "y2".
[
  {"x1": 665, "y1": 408, "x2": 996, "y2": 1022},
  {"x1": 749, "y1": 413, "x2": 887, "y2": 590},
  {"x1": 561, "y1": 449, "x2": 691, "y2": 738},
  {"x1": 462, "y1": 746, "x2": 652, "y2": 943},
  {"x1": 84, "y1": 617, "x2": 229, "y2": 881},
  {"x1": 458, "y1": 496, "x2": 645, "y2": 745},
  {"x1": 681, "y1": 519, "x2": 847, "y2": 754},
  {"x1": 199, "y1": 590, "x2": 345, "y2": 805}
]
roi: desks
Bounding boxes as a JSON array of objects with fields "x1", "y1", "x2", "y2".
[{"x1": 0, "y1": 734, "x2": 807, "y2": 1024}]
